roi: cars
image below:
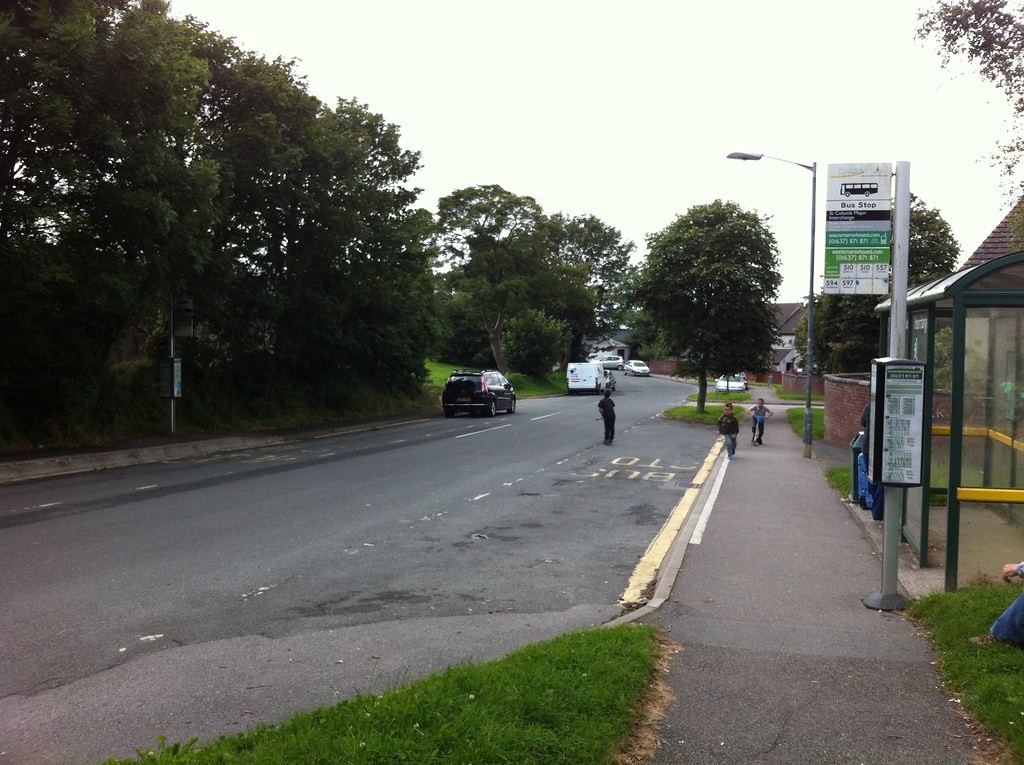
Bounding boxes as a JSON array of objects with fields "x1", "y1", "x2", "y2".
[
  {"x1": 624, "y1": 360, "x2": 651, "y2": 377},
  {"x1": 603, "y1": 370, "x2": 616, "y2": 391},
  {"x1": 714, "y1": 374, "x2": 748, "y2": 392},
  {"x1": 441, "y1": 369, "x2": 517, "y2": 418},
  {"x1": 589, "y1": 355, "x2": 624, "y2": 370},
  {"x1": 738, "y1": 370, "x2": 748, "y2": 381}
]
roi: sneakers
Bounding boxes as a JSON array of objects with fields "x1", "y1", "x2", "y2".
[{"x1": 970, "y1": 634, "x2": 998, "y2": 646}]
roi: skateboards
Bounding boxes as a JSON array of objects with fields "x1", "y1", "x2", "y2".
[{"x1": 604, "y1": 438, "x2": 613, "y2": 445}]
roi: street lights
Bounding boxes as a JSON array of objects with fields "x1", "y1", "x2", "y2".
[{"x1": 727, "y1": 151, "x2": 817, "y2": 458}]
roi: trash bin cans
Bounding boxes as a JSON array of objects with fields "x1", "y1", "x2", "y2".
[{"x1": 848, "y1": 432, "x2": 873, "y2": 509}]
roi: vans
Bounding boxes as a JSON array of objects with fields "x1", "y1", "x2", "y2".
[{"x1": 567, "y1": 362, "x2": 606, "y2": 396}]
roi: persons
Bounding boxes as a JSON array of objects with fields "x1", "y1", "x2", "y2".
[
  {"x1": 747, "y1": 399, "x2": 774, "y2": 444},
  {"x1": 597, "y1": 389, "x2": 615, "y2": 445},
  {"x1": 861, "y1": 403, "x2": 870, "y2": 489},
  {"x1": 718, "y1": 403, "x2": 739, "y2": 459},
  {"x1": 969, "y1": 560, "x2": 1024, "y2": 645}
]
